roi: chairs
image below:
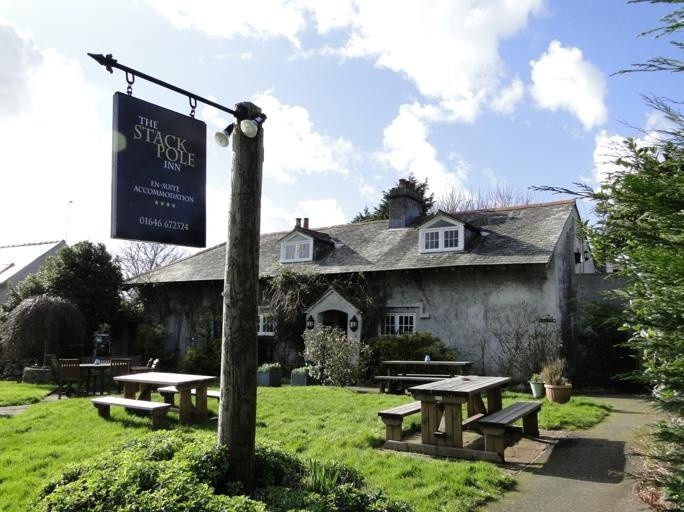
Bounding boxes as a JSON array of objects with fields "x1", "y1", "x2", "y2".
[{"x1": 45, "y1": 355, "x2": 160, "y2": 399}]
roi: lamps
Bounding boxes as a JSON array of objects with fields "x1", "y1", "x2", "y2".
[
  {"x1": 584, "y1": 251, "x2": 589, "y2": 261},
  {"x1": 350, "y1": 315, "x2": 358, "y2": 331},
  {"x1": 306, "y1": 315, "x2": 314, "y2": 329}
]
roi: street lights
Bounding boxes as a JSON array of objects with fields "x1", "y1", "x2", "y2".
[{"x1": 214, "y1": 102, "x2": 267, "y2": 487}]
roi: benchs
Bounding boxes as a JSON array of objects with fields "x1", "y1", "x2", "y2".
[
  {"x1": 157, "y1": 386, "x2": 219, "y2": 412},
  {"x1": 397, "y1": 373, "x2": 460, "y2": 378},
  {"x1": 477, "y1": 402, "x2": 543, "y2": 464},
  {"x1": 378, "y1": 401, "x2": 422, "y2": 450},
  {"x1": 373, "y1": 375, "x2": 447, "y2": 393},
  {"x1": 91, "y1": 395, "x2": 172, "y2": 431}
]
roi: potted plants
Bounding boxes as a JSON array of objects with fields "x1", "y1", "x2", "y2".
[
  {"x1": 542, "y1": 356, "x2": 572, "y2": 403},
  {"x1": 528, "y1": 373, "x2": 546, "y2": 399}
]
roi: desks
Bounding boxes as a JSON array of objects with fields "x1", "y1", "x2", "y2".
[
  {"x1": 112, "y1": 372, "x2": 219, "y2": 427},
  {"x1": 382, "y1": 376, "x2": 513, "y2": 464},
  {"x1": 383, "y1": 360, "x2": 473, "y2": 395}
]
